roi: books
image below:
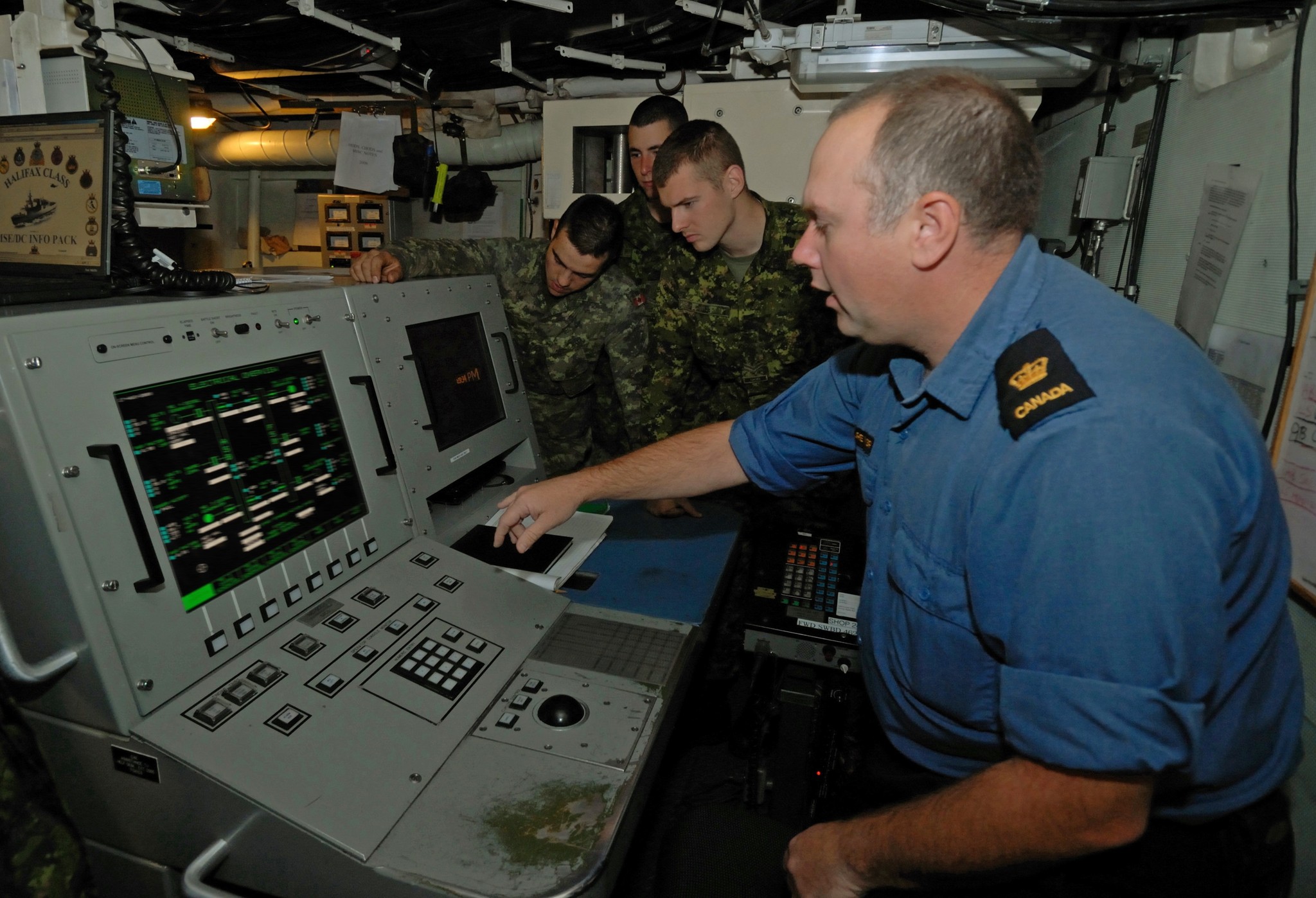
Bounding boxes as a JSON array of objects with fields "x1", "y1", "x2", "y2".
[
  {"x1": 450, "y1": 523, "x2": 576, "y2": 574},
  {"x1": 486, "y1": 500, "x2": 614, "y2": 593}
]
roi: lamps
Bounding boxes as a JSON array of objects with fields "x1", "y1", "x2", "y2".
[{"x1": 190, "y1": 99, "x2": 220, "y2": 130}]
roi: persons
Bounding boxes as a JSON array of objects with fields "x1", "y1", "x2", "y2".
[
  {"x1": 640, "y1": 117, "x2": 831, "y2": 518},
  {"x1": 602, "y1": 93, "x2": 692, "y2": 366},
  {"x1": 353, "y1": 192, "x2": 704, "y2": 518},
  {"x1": 519, "y1": 64, "x2": 1303, "y2": 898}
]
